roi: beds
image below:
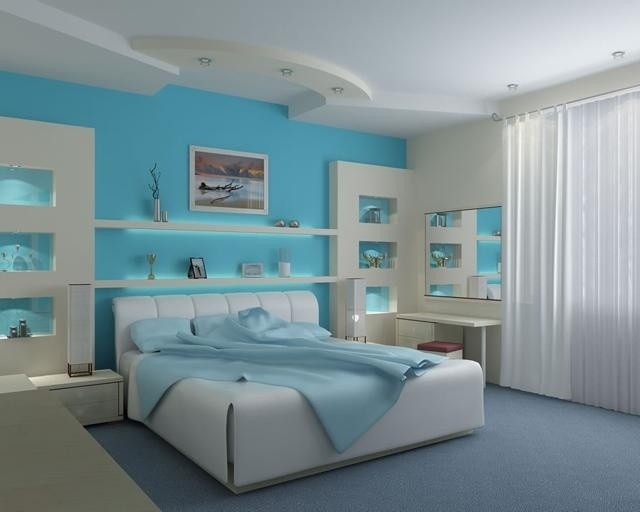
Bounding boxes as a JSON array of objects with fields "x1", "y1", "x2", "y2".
[{"x1": 113, "y1": 290, "x2": 485, "y2": 495}]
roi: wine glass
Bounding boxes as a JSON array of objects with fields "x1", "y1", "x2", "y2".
[{"x1": 145, "y1": 253, "x2": 156, "y2": 280}]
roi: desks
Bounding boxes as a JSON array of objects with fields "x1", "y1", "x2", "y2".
[{"x1": 395, "y1": 313, "x2": 504, "y2": 388}]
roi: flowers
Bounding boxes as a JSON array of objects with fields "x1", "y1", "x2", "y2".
[
  {"x1": 362, "y1": 248, "x2": 383, "y2": 259},
  {"x1": 432, "y1": 248, "x2": 444, "y2": 262}
]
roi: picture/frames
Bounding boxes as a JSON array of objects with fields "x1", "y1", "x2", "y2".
[
  {"x1": 242, "y1": 263, "x2": 263, "y2": 276},
  {"x1": 190, "y1": 257, "x2": 207, "y2": 278},
  {"x1": 188, "y1": 144, "x2": 269, "y2": 215}
]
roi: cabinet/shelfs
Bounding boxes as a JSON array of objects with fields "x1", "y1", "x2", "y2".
[
  {"x1": 94, "y1": 219, "x2": 336, "y2": 288},
  {"x1": 0, "y1": 116, "x2": 95, "y2": 376},
  {"x1": 426, "y1": 209, "x2": 500, "y2": 295},
  {"x1": 328, "y1": 160, "x2": 415, "y2": 342}
]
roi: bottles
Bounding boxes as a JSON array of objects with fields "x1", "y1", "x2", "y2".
[
  {"x1": 9, "y1": 326, "x2": 17, "y2": 337},
  {"x1": 19, "y1": 320, "x2": 26, "y2": 336}
]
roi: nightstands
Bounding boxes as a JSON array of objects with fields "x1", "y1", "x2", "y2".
[{"x1": 29, "y1": 368, "x2": 124, "y2": 426}]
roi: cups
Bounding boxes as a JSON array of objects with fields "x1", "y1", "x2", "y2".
[
  {"x1": 277, "y1": 261, "x2": 290, "y2": 277},
  {"x1": 162, "y1": 210, "x2": 168, "y2": 222}
]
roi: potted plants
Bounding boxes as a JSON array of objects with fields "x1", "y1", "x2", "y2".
[{"x1": 148, "y1": 162, "x2": 161, "y2": 222}]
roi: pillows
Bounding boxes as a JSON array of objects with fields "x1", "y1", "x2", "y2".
[
  {"x1": 290, "y1": 321, "x2": 332, "y2": 339},
  {"x1": 129, "y1": 318, "x2": 194, "y2": 352},
  {"x1": 191, "y1": 315, "x2": 236, "y2": 336}
]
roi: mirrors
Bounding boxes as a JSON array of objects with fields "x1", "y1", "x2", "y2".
[{"x1": 424, "y1": 206, "x2": 502, "y2": 302}]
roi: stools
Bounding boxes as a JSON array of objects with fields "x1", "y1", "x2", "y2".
[{"x1": 418, "y1": 341, "x2": 463, "y2": 360}]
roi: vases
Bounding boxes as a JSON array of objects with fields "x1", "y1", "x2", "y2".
[
  {"x1": 438, "y1": 259, "x2": 445, "y2": 267},
  {"x1": 369, "y1": 257, "x2": 378, "y2": 267}
]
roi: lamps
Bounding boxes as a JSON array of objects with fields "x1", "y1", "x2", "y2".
[
  {"x1": 332, "y1": 86, "x2": 343, "y2": 94},
  {"x1": 66, "y1": 282, "x2": 95, "y2": 378},
  {"x1": 280, "y1": 68, "x2": 292, "y2": 77},
  {"x1": 345, "y1": 277, "x2": 366, "y2": 343},
  {"x1": 507, "y1": 83, "x2": 518, "y2": 91},
  {"x1": 612, "y1": 51, "x2": 623, "y2": 61},
  {"x1": 198, "y1": 57, "x2": 210, "y2": 65}
]
